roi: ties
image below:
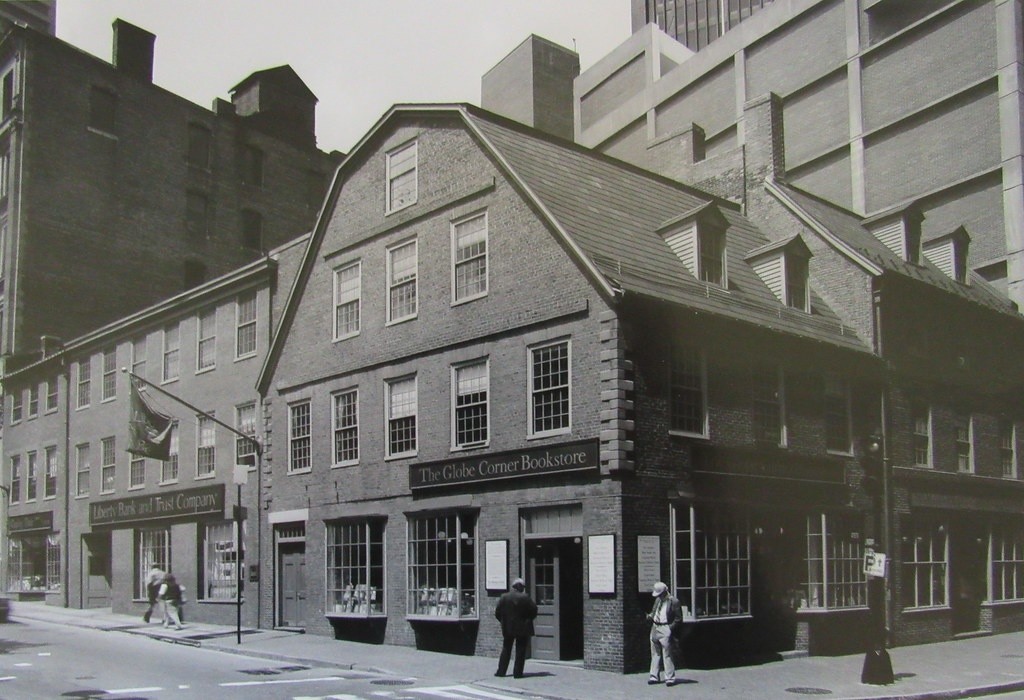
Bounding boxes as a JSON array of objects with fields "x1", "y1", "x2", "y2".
[{"x1": 656, "y1": 600, "x2": 663, "y2": 624}]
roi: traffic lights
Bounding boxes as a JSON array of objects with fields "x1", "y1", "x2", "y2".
[{"x1": 859, "y1": 434, "x2": 887, "y2": 501}]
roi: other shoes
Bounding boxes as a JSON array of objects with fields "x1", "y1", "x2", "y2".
[
  {"x1": 143, "y1": 617, "x2": 149, "y2": 623},
  {"x1": 174, "y1": 627, "x2": 182, "y2": 630},
  {"x1": 666, "y1": 681, "x2": 673, "y2": 686},
  {"x1": 648, "y1": 680, "x2": 659, "y2": 684},
  {"x1": 494, "y1": 673, "x2": 505, "y2": 677}
]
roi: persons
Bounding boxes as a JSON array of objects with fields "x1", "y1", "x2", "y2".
[
  {"x1": 156, "y1": 572, "x2": 186, "y2": 631},
  {"x1": 645, "y1": 581, "x2": 682, "y2": 686},
  {"x1": 495, "y1": 578, "x2": 538, "y2": 679},
  {"x1": 143, "y1": 562, "x2": 169, "y2": 623}
]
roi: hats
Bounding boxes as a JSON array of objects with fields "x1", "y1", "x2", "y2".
[
  {"x1": 512, "y1": 578, "x2": 526, "y2": 588},
  {"x1": 652, "y1": 582, "x2": 667, "y2": 597}
]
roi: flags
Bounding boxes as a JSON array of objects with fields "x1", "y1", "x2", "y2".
[{"x1": 124, "y1": 385, "x2": 172, "y2": 462}]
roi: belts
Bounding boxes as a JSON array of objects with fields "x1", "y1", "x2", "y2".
[{"x1": 653, "y1": 621, "x2": 668, "y2": 625}]
